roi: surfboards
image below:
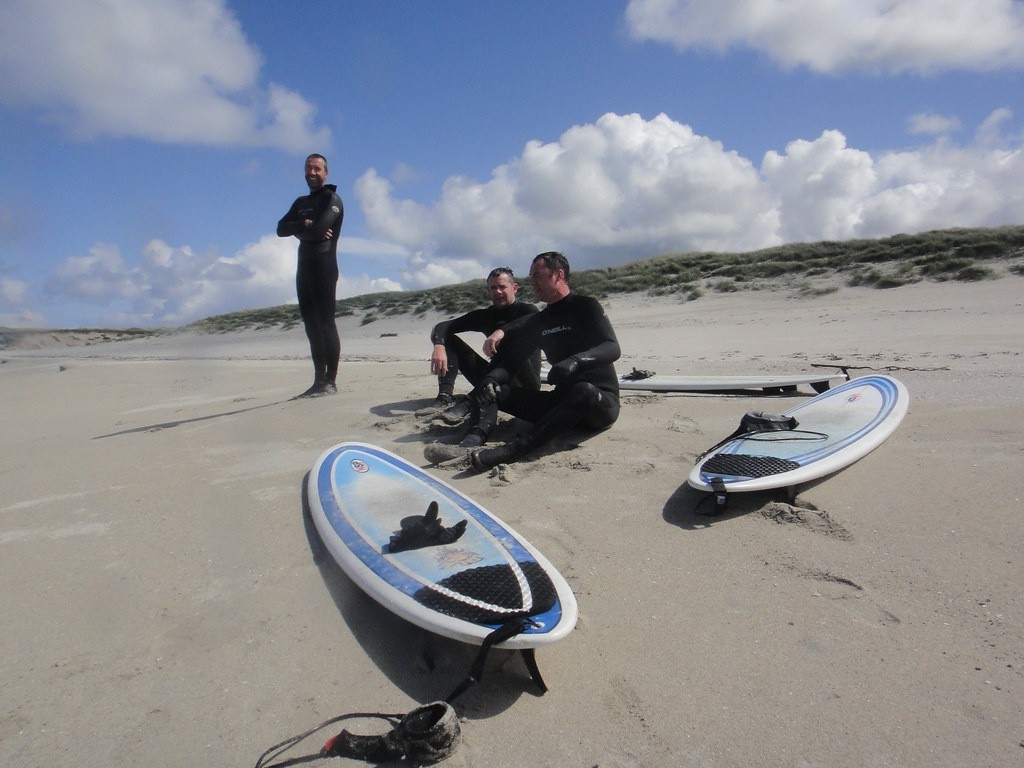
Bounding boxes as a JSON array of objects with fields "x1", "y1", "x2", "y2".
[
  {"x1": 307, "y1": 441, "x2": 579, "y2": 650},
  {"x1": 540, "y1": 367, "x2": 849, "y2": 390},
  {"x1": 688, "y1": 373, "x2": 910, "y2": 493}
]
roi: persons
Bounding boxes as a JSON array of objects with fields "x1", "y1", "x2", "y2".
[
  {"x1": 277, "y1": 154, "x2": 344, "y2": 398},
  {"x1": 414, "y1": 268, "x2": 541, "y2": 425},
  {"x1": 459, "y1": 251, "x2": 622, "y2": 471}
]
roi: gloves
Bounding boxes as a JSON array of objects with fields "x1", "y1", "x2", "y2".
[
  {"x1": 547, "y1": 359, "x2": 579, "y2": 385},
  {"x1": 477, "y1": 378, "x2": 501, "y2": 405},
  {"x1": 388, "y1": 501, "x2": 468, "y2": 553}
]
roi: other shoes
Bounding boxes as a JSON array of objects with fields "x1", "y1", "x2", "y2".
[
  {"x1": 440, "y1": 397, "x2": 474, "y2": 425},
  {"x1": 424, "y1": 435, "x2": 478, "y2": 466},
  {"x1": 414, "y1": 394, "x2": 457, "y2": 416},
  {"x1": 299, "y1": 382, "x2": 337, "y2": 397},
  {"x1": 471, "y1": 442, "x2": 522, "y2": 473}
]
